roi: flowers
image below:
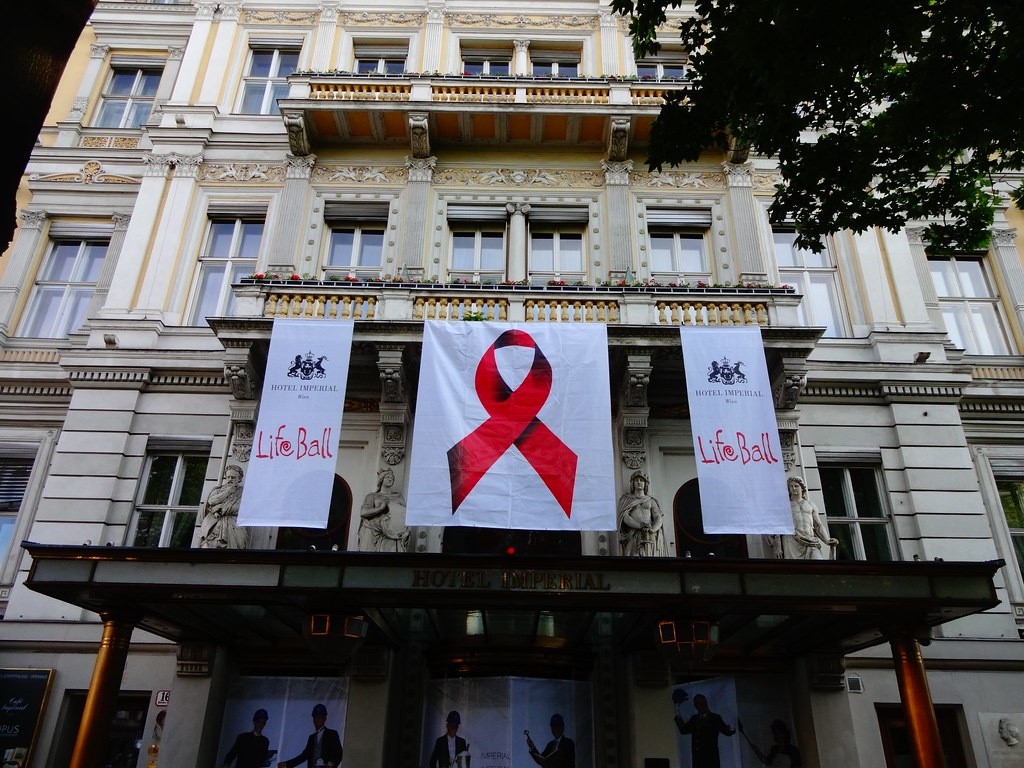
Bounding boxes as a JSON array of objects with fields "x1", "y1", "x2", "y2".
[
  {"x1": 289, "y1": 65, "x2": 688, "y2": 82},
  {"x1": 250, "y1": 272, "x2": 797, "y2": 297}
]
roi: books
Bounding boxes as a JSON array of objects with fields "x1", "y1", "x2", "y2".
[{"x1": 534, "y1": 750, "x2": 558, "y2": 764}]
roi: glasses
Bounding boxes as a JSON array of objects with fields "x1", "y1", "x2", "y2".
[{"x1": 257, "y1": 720, "x2": 265, "y2": 723}]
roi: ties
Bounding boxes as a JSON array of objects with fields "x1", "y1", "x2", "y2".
[
  {"x1": 700, "y1": 713, "x2": 705, "y2": 720},
  {"x1": 552, "y1": 741, "x2": 558, "y2": 751}
]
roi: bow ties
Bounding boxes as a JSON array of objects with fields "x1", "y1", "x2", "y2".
[{"x1": 315, "y1": 727, "x2": 324, "y2": 735}]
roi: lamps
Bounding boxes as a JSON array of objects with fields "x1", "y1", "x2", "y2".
[
  {"x1": 658, "y1": 619, "x2": 722, "y2": 662},
  {"x1": 307, "y1": 613, "x2": 364, "y2": 646}
]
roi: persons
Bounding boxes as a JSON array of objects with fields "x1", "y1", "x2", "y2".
[
  {"x1": 358, "y1": 468, "x2": 411, "y2": 553},
  {"x1": 197, "y1": 465, "x2": 250, "y2": 548},
  {"x1": 998, "y1": 718, "x2": 1020, "y2": 745},
  {"x1": 673, "y1": 687, "x2": 736, "y2": 768},
  {"x1": 219, "y1": 709, "x2": 277, "y2": 768},
  {"x1": 617, "y1": 470, "x2": 669, "y2": 557},
  {"x1": 524, "y1": 713, "x2": 577, "y2": 768},
  {"x1": 737, "y1": 717, "x2": 802, "y2": 768},
  {"x1": 277, "y1": 704, "x2": 343, "y2": 768},
  {"x1": 429, "y1": 711, "x2": 471, "y2": 768},
  {"x1": 763, "y1": 475, "x2": 839, "y2": 560}
]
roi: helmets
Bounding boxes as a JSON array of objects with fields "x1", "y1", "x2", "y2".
[
  {"x1": 770, "y1": 719, "x2": 788, "y2": 732},
  {"x1": 672, "y1": 688, "x2": 689, "y2": 707},
  {"x1": 550, "y1": 714, "x2": 563, "y2": 726},
  {"x1": 312, "y1": 704, "x2": 327, "y2": 716},
  {"x1": 447, "y1": 711, "x2": 460, "y2": 724},
  {"x1": 253, "y1": 709, "x2": 268, "y2": 720}
]
